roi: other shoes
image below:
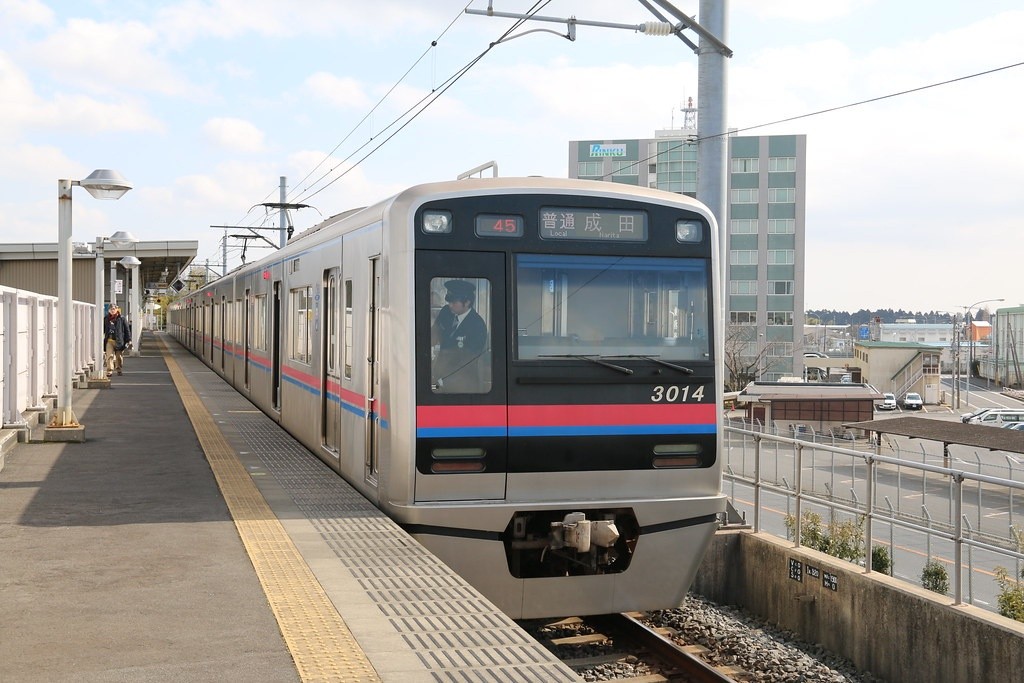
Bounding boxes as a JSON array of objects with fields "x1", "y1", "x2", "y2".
[
  {"x1": 106, "y1": 371, "x2": 113, "y2": 377},
  {"x1": 117, "y1": 371, "x2": 122, "y2": 375}
]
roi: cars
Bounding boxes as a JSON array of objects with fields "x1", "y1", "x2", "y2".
[
  {"x1": 878, "y1": 394, "x2": 897, "y2": 409},
  {"x1": 960, "y1": 407, "x2": 1024, "y2": 431},
  {"x1": 803, "y1": 351, "x2": 829, "y2": 359},
  {"x1": 840, "y1": 375, "x2": 852, "y2": 382},
  {"x1": 903, "y1": 392, "x2": 924, "y2": 410}
]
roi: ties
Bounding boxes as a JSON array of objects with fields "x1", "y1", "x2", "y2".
[{"x1": 448, "y1": 314, "x2": 459, "y2": 336}]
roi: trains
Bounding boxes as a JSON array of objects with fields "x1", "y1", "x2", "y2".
[{"x1": 167, "y1": 175, "x2": 729, "y2": 621}]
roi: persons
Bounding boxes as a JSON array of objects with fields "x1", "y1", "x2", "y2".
[
  {"x1": 103, "y1": 303, "x2": 132, "y2": 377},
  {"x1": 431, "y1": 280, "x2": 487, "y2": 405}
]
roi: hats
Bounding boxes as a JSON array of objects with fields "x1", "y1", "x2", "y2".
[
  {"x1": 106, "y1": 303, "x2": 118, "y2": 310},
  {"x1": 443, "y1": 280, "x2": 476, "y2": 300}
]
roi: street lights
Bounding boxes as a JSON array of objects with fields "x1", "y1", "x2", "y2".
[
  {"x1": 90, "y1": 230, "x2": 139, "y2": 380},
  {"x1": 731, "y1": 403, "x2": 736, "y2": 411},
  {"x1": 965, "y1": 298, "x2": 1006, "y2": 408},
  {"x1": 45, "y1": 170, "x2": 135, "y2": 428},
  {"x1": 109, "y1": 255, "x2": 143, "y2": 307},
  {"x1": 810, "y1": 312, "x2": 830, "y2": 352}
]
